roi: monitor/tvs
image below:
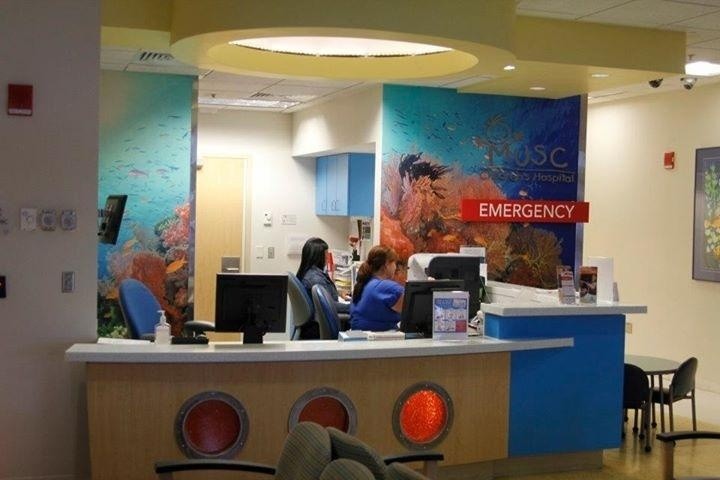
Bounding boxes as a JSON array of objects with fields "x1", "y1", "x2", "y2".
[
  {"x1": 215, "y1": 269, "x2": 289, "y2": 345},
  {"x1": 400, "y1": 279, "x2": 470, "y2": 341}
]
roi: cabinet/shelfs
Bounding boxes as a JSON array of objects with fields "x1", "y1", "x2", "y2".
[{"x1": 315, "y1": 152, "x2": 375, "y2": 217}]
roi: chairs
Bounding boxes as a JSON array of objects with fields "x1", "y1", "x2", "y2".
[
  {"x1": 311, "y1": 284, "x2": 424, "y2": 340},
  {"x1": 283, "y1": 271, "x2": 350, "y2": 341},
  {"x1": 154, "y1": 421, "x2": 444, "y2": 480},
  {"x1": 622, "y1": 354, "x2": 698, "y2": 452},
  {"x1": 656, "y1": 431, "x2": 719, "y2": 480},
  {"x1": 117, "y1": 278, "x2": 216, "y2": 344}
]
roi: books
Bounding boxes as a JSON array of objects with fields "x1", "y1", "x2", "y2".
[{"x1": 338, "y1": 330, "x2": 405, "y2": 342}]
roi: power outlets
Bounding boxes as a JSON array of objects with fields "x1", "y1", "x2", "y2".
[{"x1": 62, "y1": 271, "x2": 75, "y2": 294}]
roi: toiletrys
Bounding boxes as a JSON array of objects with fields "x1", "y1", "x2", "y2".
[{"x1": 154, "y1": 310, "x2": 172, "y2": 346}]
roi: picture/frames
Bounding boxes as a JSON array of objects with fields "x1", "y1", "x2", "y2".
[{"x1": 692, "y1": 146, "x2": 720, "y2": 282}]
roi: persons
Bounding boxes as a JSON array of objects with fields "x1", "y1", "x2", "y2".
[
  {"x1": 349, "y1": 245, "x2": 405, "y2": 331},
  {"x1": 297, "y1": 238, "x2": 350, "y2": 339}
]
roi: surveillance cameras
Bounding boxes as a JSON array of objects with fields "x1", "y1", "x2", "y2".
[{"x1": 680, "y1": 75, "x2": 697, "y2": 90}]
